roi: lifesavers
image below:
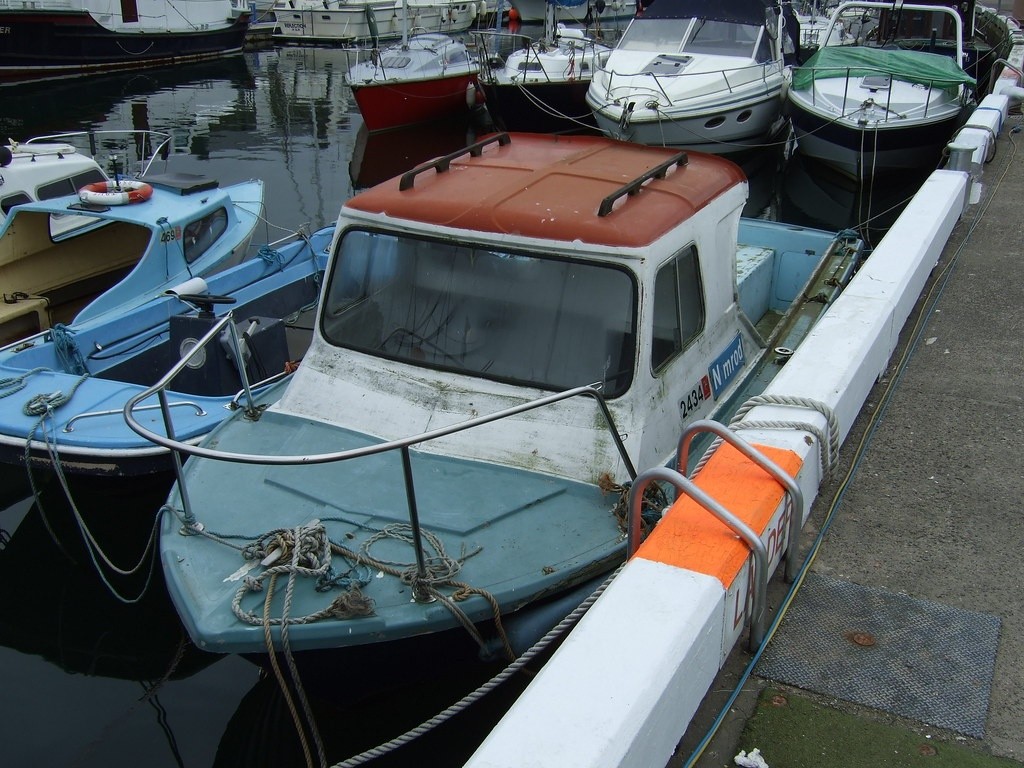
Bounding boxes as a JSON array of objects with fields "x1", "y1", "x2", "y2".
[{"x1": 78, "y1": 179, "x2": 154, "y2": 205}]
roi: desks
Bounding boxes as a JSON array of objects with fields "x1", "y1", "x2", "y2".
[{"x1": 0, "y1": 294, "x2": 53, "y2": 332}]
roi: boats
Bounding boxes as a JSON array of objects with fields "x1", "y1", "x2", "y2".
[
  {"x1": 1, "y1": 213, "x2": 397, "y2": 498},
  {"x1": 465, "y1": 0, "x2": 617, "y2": 132},
  {"x1": 343, "y1": 29, "x2": 498, "y2": 131},
  {"x1": 0, "y1": 0, "x2": 251, "y2": 104},
  {"x1": 0, "y1": 128, "x2": 172, "y2": 231},
  {"x1": 824, "y1": 1, "x2": 869, "y2": 21},
  {"x1": 340, "y1": 116, "x2": 493, "y2": 194},
  {"x1": 475, "y1": 0, "x2": 638, "y2": 24},
  {"x1": 1, "y1": 175, "x2": 265, "y2": 359},
  {"x1": 158, "y1": 122, "x2": 863, "y2": 663},
  {"x1": 583, "y1": 0, "x2": 856, "y2": 153},
  {"x1": 794, "y1": 11, "x2": 857, "y2": 63},
  {"x1": 271, "y1": 0, "x2": 484, "y2": 47},
  {"x1": 787, "y1": 2, "x2": 979, "y2": 198},
  {"x1": 246, "y1": 5, "x2": 282, "y2": 42}
]
